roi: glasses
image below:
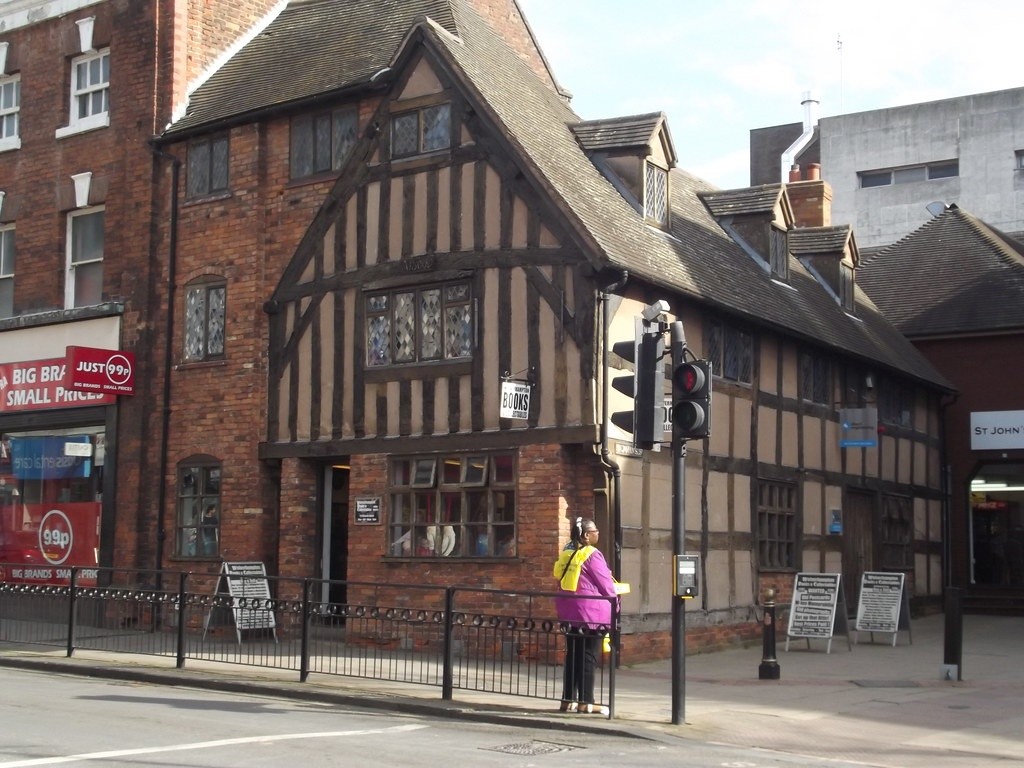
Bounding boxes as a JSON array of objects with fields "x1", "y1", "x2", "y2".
[{"x1": 588, "y1": 529, "x2": 598, "y2": 532}]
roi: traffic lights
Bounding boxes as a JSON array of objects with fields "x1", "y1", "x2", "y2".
[
  {"x1": 610, "y1": 314, "x2": 683, "y2": 459},
  {"x1": 672, "y1": 357, "x2": 711, "y2": 440}
]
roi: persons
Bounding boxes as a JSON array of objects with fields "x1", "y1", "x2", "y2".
[
  {"x1": 203, "y1": 504, "x2": 218, "y2": 538},
  {"x1": 553, "y1": 517, "x2": 619, "y2": 713}
]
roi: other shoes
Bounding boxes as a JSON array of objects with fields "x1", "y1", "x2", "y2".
[
  {"x1": 560, "y1": 702, "x2": 577, "y2": 711},
  {"x1": 577, "y1": 704, "x2": 602, "y2": 714}
]
